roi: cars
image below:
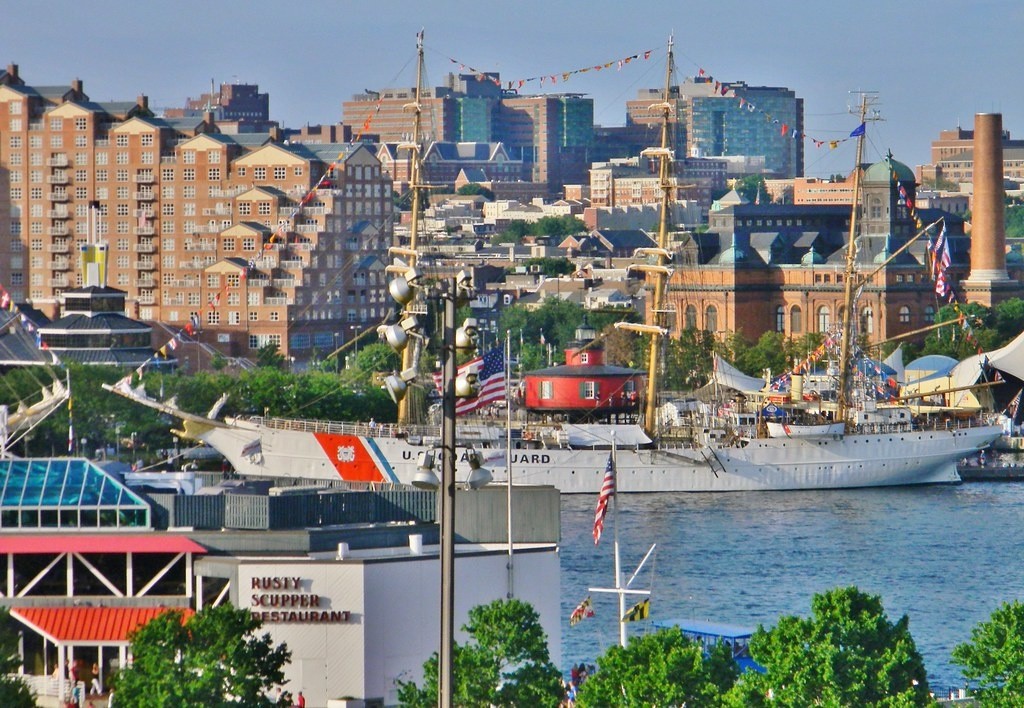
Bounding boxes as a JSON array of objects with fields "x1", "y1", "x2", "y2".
[
  {"x1": 830, "y1": 366, "x2": 839, "y2": 373},
  {"x1": 802, "y1": 390, "x2": 821, "y2": 401}
]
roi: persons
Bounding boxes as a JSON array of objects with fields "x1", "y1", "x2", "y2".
[
  {"x1": 962, "y1": 450, "x2": 987, "y2": 467},
  {"x1": 558, "y1": 660, "x2": 595, "y2": 708},
  {"x1": 52, "y1": 660, "x2": 116, "y2": 708},
  {"x1": 276, "y1": 688, "x2": 305, "y2": 708},
  {"x1": 369, "y1": 417, "x2": 376, "y2": 436}
]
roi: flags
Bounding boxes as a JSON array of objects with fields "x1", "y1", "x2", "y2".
[
  {"x1": 593, "y1": 452, "x2": 613, "y2": 544},
  {"x1": 241, "y1": 439, "x2": 262, "y2": 457},
  {"x1": 428, "y1": 42, "x2": 865, "y2": 158},
  {"x1": 622, "y1": 598, "x2": 649, "y2": 622},
  {"x1": 1010, "y1": 390, "x2": 1022, "y2": 418},
  {"x1": 431, "y1": 342, "x2": 505, "y2": 415},
  {"x1": 886, "y1": 154, "x2": 1007, "y2": 383},
  {"x1": 0, "y1": 283, "x2": 65, "y2": 370},
  {"x1": 123, "y1": 88, "x2": 386, "y2": 386},
  {"x1": 570, "y1": 596, "x2": 594, "y2": 626},
  {"x1": 768, "y1": 328, "x2": 904, "y2": 405}
]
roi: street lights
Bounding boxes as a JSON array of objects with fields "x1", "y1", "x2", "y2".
[
  {"x1": 172, "y1": 435, "x2": 178, "y2": 471},
  {"x1": 81, "y1": 437, "x2": 87, "y2": 457},
  {"x1": 1010, "y1": 404, "x2": 1015, "y2": 437},
  {"x1": 946, "y1": 371, "x2": 952, "y2": 407},
  {"x1": 385, "y1": 269, "x2": 481, "y2": 708},
  {"x1": 184, "y1": 460, "x2": 199, "y2": 473},
  {"x1": 863, "y1": 359, "x2": 867, "y2": 377}
]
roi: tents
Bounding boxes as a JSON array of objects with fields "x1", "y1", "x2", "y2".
[{"x1": 756, "y1": 402, "x2": 786, "y2": 417}]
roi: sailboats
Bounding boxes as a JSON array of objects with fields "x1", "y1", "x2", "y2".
[
  {"x1": 101, "y1": 26, "x2": 1003, "y2": 493},
  {"x1": 1, "y1": 285, "x2": 72, "y2": 434}
]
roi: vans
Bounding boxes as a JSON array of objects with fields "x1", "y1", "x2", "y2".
[{"x1": 193, "y1": 480, "x2": 275, "y2": 495}]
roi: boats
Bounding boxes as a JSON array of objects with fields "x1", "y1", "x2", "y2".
[
  {"x1": 764, "y1": 410, "x2": 845, "y2": 437},
  {"x1": 647, "y1": 617, "x2": 766, "y2": 676}
]
